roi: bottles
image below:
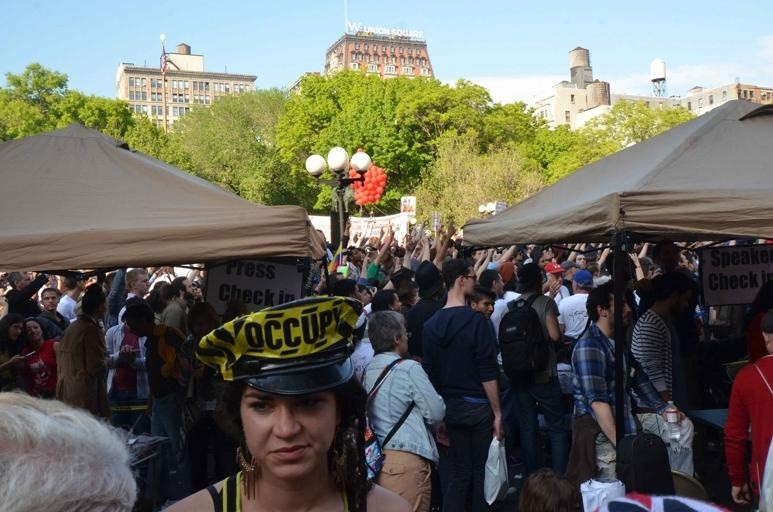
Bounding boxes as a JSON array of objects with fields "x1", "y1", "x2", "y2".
[{"x1": 665, "y1": 400, "x2": 684, "y2": 443}]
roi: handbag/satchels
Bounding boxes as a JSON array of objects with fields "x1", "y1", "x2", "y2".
[{"x1": 614, "y1": 431, "x2": 678, "y2": 498}]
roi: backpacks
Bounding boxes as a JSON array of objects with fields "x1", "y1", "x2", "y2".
[
  {"x1": 337, "y1": 356, "x2": 416, "y2": 486},
  {"x1": 497, "y1": 293, "x2": 547, "y2": 381}
]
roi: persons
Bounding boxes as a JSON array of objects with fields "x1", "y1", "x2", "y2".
[{"x1": 0, "y1": 221, "x2": 773, "y2": 511}]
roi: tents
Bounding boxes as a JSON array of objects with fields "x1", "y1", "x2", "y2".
[{"x1": 0, "y1": 129, "x2": 326, "y2": 296}]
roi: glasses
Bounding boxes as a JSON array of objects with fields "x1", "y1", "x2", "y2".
[
  {"x1": 400, "y1": 332, "x2": 412, "y2": 338},
  {"x1": 456, "y1": 273, "x2": 477, "y2": 280}
]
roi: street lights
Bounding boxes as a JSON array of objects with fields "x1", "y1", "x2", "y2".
[
  {"x1": 303, "y1": 145, "x2": 377, "y2": 264},
  {"x1": 477, "y1": 200, "x2": 497, "y2": 217}
]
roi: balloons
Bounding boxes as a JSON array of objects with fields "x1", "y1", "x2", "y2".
[{"x1": 349, "y1": 148, "x2": 388, "y2": 205}]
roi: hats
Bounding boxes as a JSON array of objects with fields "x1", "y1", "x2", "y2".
[
  {"x1": 191, "y1": 293, "x2": 364, "y2": 396},
  {"x1": 414, "y1": 259, "x2": 443, "y2": 301},
  {"x1": 543, "y1": 261, "x2": 566, "y2": 274},
  {"x1": 570, "y1": 269, "x2": 593, "y2": 285},
  {"x1": 513, "y1": 263, "x2": 541, "y2": 291}
]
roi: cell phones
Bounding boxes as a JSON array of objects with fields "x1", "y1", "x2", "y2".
[{"x1": 20, "y1": 351, "x2": 36, "y2": 357}]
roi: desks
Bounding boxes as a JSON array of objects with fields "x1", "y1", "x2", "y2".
[{"x1": 684, "y1": 408, "x2": 752, "y2": 497}]
